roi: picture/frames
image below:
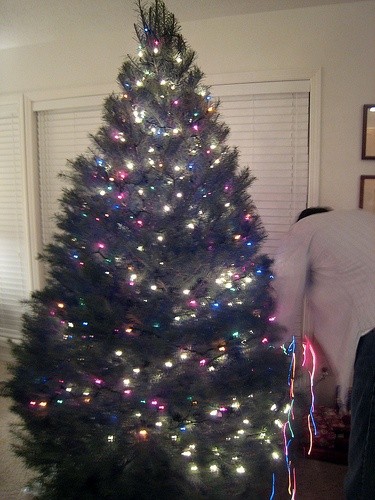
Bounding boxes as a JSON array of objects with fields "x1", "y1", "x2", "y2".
[
  {"x1": 357, "y1": 175, "x2": 375, "y2": 211},
  {"x1": 361, "y1": 103, "x2": 375, "y2": 160}
]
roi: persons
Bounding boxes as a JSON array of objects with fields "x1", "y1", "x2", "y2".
[{"x1": 270, "y1": 204, "x2": 374, "y2": 500}]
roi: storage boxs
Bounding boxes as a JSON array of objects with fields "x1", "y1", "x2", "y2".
[{"x1": 295, "y1": 404, "x2": 353, "y2": 459}]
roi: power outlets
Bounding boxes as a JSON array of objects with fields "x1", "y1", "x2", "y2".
[{"x1": 315, "y1": 363, "x2": 331, "y2": 377}]
